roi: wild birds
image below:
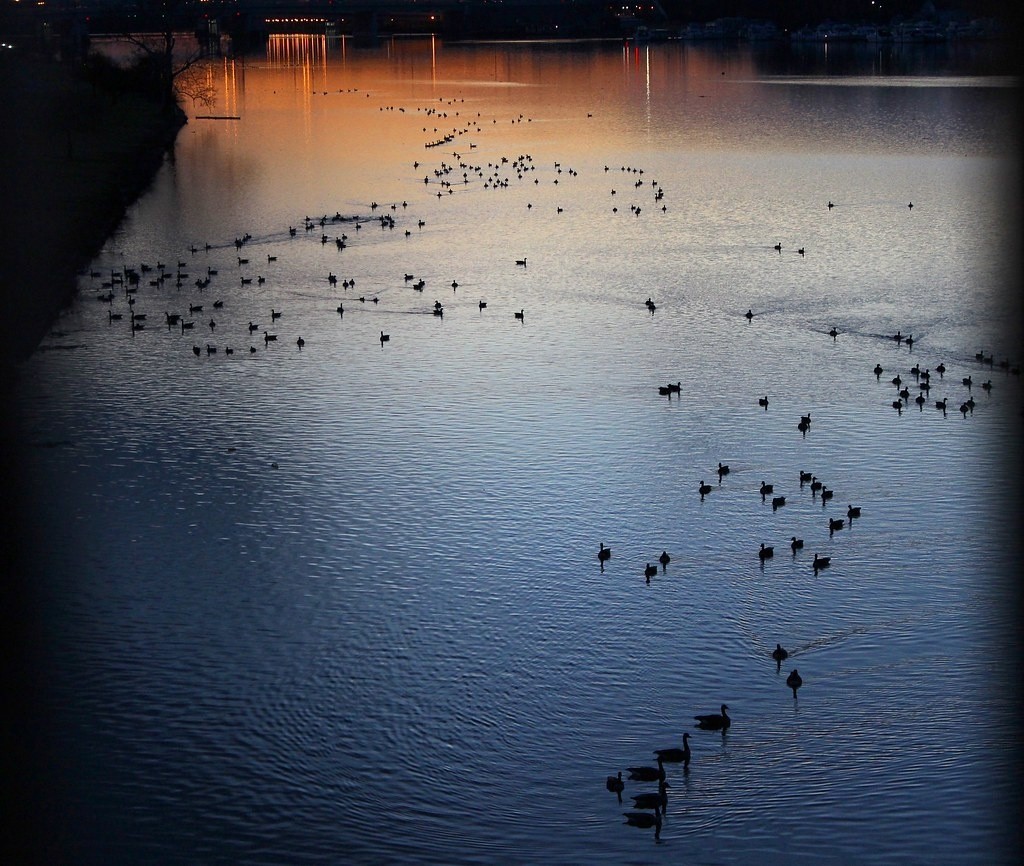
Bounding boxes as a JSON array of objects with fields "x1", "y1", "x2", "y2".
[
  {"x1": 644, "y1": 197, "x2": 1019, "y2": 438},
  {"x1": 269, "y1": 82, "x2": 669, "y2": 219},
  {"x1": 86, "y1": 214, "x2": 532, "y2": 360},
  {"x1": 595, "y1": 461, "x2": 862, "y2": 837}
]
